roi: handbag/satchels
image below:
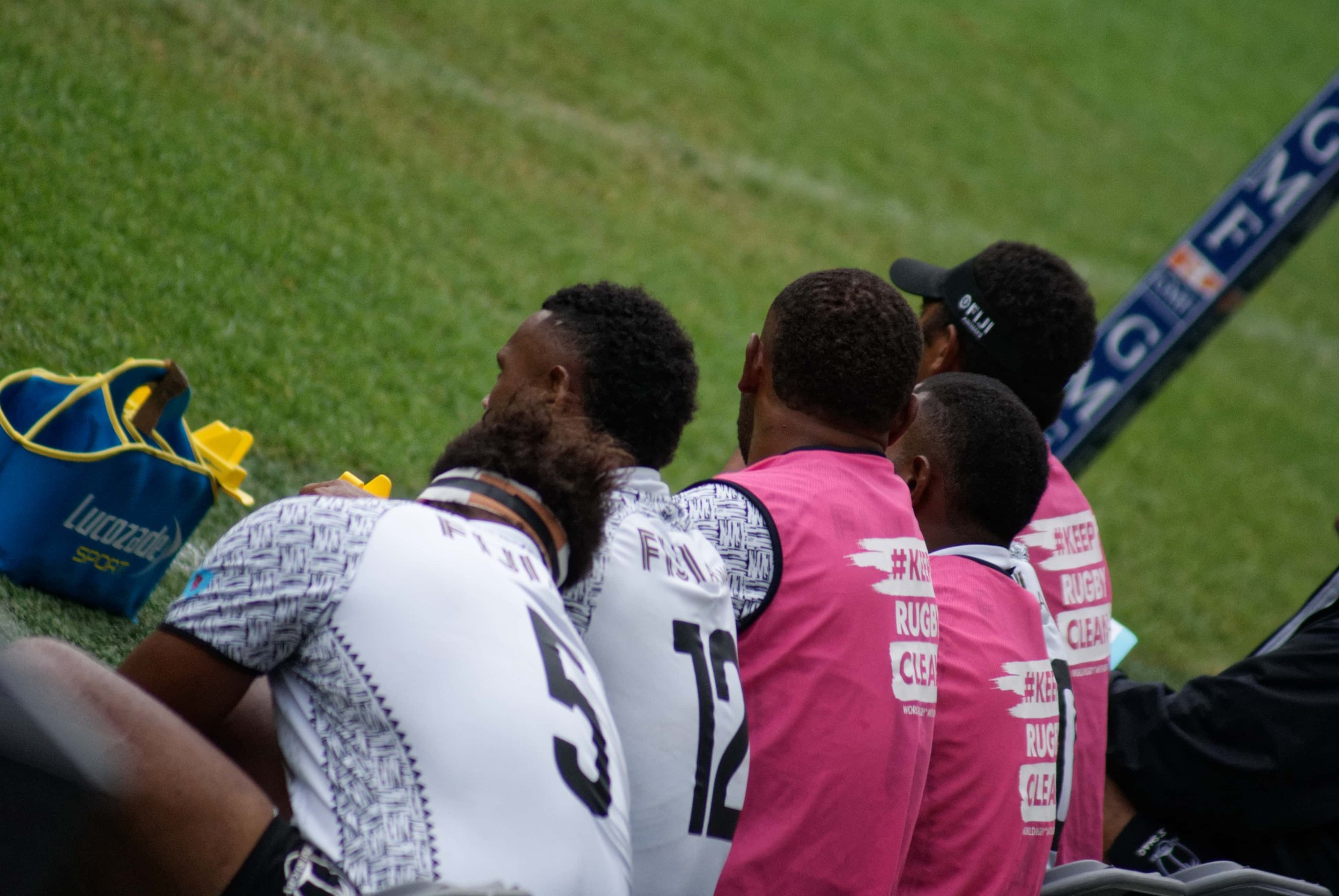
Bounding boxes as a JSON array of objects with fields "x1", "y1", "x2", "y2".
[{"x1": 1, "y1": 360, "x2": 255, "y2": 621}]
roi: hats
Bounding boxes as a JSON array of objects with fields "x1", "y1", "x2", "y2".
[{"x1": 891, "y1": 253, "x2": 1082, "y2": 390}]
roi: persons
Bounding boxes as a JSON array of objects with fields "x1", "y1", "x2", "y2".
[
  {"x1": 298, "y1": 281, "x2": 750, "y2": 895},
  {"x1": 0, "y1": 404, "x2": 631, "y2": 896},
  {"x1": 889, "y1": 240, "x2": 1111, "y2": 870},
  {"x1": 885, "y1": 371, "x2": 1076, "y2": 896},
  {"x1": 1103, "y1": 567, "x2": 1339, "y2": 896},
  {"x1": 672, "y1": 267, "x2": 939, "y2": 896}
]
